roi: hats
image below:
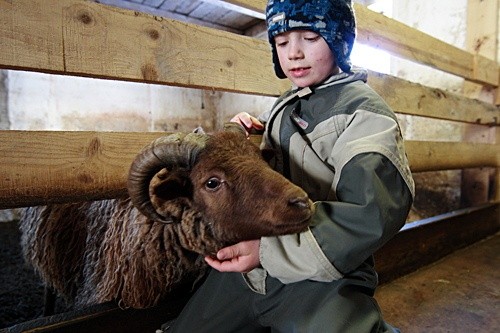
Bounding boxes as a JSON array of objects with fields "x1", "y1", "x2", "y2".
[{"x1": 266, "y1": 0, "x2": 356, "y2": 79}]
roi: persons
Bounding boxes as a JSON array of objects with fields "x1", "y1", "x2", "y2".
[{"x1": 163, "y1": 0, "x2": 416, "y2": 333}]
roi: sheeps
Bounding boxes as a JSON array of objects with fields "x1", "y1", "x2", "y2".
[{"x1": 14, "y1": 121, "x2": 317, "y2": 318}]
